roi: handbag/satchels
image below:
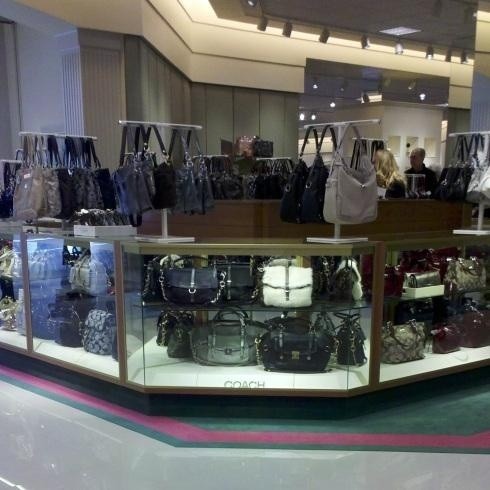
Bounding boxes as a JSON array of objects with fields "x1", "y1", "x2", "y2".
[
  {"x1": 382, "y1": 247, "x2": 490, "y2": 364},
  {"x1": 430, "y1": 166, "x2": 490, "y2": 205},
  {"x1": 279, "y1": 155, "x2": 378, "y2": 224},
  {"x1": 234, "y1": 139, "x2": 273, "y2": 158},
  {"x1": 141, "y1": 254, "x2": 366, "y2": 373},
  {"x1": 0, "y1": 251, "x2": 116, "y2": 354},
  {"x1": 0, "y1": 158, "x2": 216, "y2": 221},
  {"x1": 211, "y1": 170, "x2": 290, "y2": 199}
]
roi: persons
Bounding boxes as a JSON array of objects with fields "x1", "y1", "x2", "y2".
[
  {"x1": 403, "y1": 146, "x2": 438, "y2": 197},
  {"x1": 370, "y1": 147, "x2": 406, "y2": 199}
]
